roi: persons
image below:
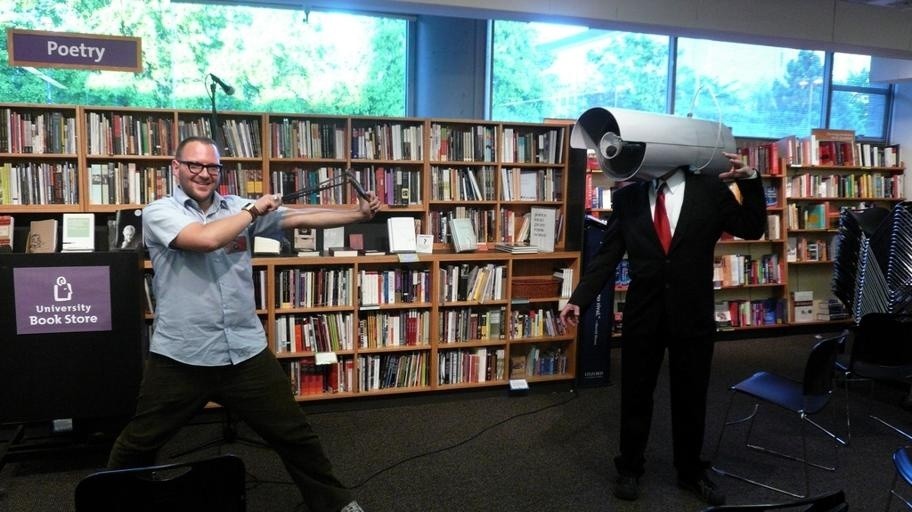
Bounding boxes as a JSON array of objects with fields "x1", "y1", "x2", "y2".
[
  {"x1": 108, "y1": 136, "x2": 381, "y2": 510},
  {"x1": 559, "y1": 152, "x2": 768, "y2": 506}
]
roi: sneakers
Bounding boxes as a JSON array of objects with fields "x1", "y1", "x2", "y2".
[
  {"x1": 612, "y1": 473, "x2": 640, "y2": 499},
  {"x1": 676, "y1": 472, "x2": 725, "y2": 505},
  {"x1": 340, "y1": 499, "x2": 365, "y2": 511}
]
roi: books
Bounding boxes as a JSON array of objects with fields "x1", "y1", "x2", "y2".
[
  {"x1": 1, "y1": 108, "x2": 95, "y2": 253},
  {"x1": 84, "y1": 112, "x2": 577, "y2": 394},
  {"x1": 714, "y1": 130, "x2": 905, "y2": 329},
  {"x1": 587, "y1": 151, "x2": 631, "y2": 335}
]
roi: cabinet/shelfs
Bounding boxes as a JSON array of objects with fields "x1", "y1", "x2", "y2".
[
  {"x1": 0, "y1": 99, "x2": 592, "y2": 409},
  {"x1": 544, "y1": 113, "x2": 907, "y2": 346}
]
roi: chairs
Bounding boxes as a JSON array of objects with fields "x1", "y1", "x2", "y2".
[
  {"x1": 74, "y1": 456, "x2": 246, "y2": 509},
  {"x1": 699, "y1": 313, "x2": 912, "y2": 512}
]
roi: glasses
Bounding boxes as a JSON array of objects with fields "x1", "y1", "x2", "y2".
[{"x1": 177, "y1": 161, "x2": 224, "y2": 176}]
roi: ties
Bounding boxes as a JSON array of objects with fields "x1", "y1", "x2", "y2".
[{"x1": 653, "y1": 183, "x2": 672, "y2": 256}]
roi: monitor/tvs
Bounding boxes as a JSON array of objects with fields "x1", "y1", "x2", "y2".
[{"x1": 0, "y1": 250, "x2": 143, "y2": 349}]
